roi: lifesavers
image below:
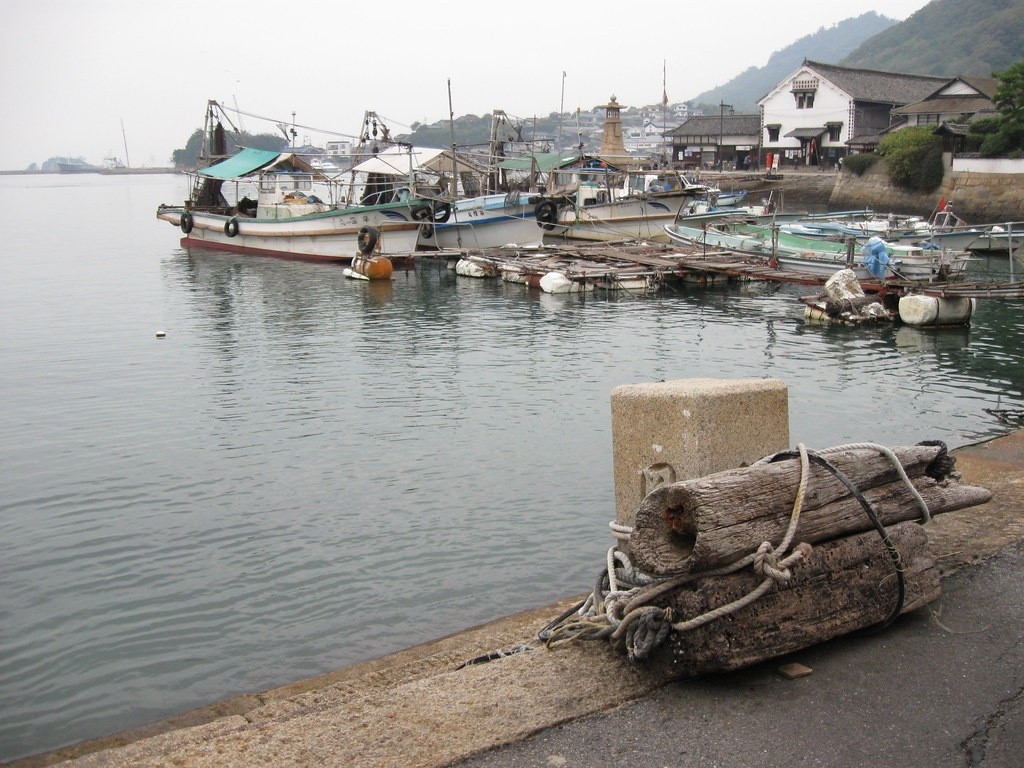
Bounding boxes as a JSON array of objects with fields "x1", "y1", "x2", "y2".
[
  {"x1": 180, "y1": 212, "x2": 194, "y2": 234},
  {"x1": 434, "y1": 205, "x2": 451, "y2": 222},
  {"x1": 537, "y1": 209, "x2": 555, "y2": 230},
  {"x1": 224, "y1": 218, "x2": 239, "y2": 237},
  {"x1": 357, "y1": 226, "x2": 376, "y2": 253},
  {"x1": 410, "y1": 204, "x2": 432, "y2": 221},
  {"x1": 535, "y1": 197, "x2": 557, "y2": 221}
]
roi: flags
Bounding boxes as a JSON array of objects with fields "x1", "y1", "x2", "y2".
[
  {"x1": 809, "y1": 138, "x2": 816, "y2": 155},
  {"x1": 938, "y1": 196, "x2": 945, "y2": 211}
]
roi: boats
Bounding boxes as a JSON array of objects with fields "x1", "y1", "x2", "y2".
[
  {"x1": 771, "y1": 203, "x2": 1024, "y2": 256},
  {"x1": 662, "y1": 174, "x2": 973, "y2": 284},
  {"x1": 155, "y1": 55, "x2": 785, "y2": 271}
]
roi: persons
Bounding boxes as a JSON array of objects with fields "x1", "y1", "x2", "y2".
[
  {"x1": 834, "y1": 157, "x2": 843, "y2": 172},
  {"x1": 716, "y1": 154, "x2": 760, "y2": 171}
]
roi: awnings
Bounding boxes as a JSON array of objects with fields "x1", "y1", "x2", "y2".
[{"x1": 783, "y1": 127, "x2": 827, "y2": 137}]
